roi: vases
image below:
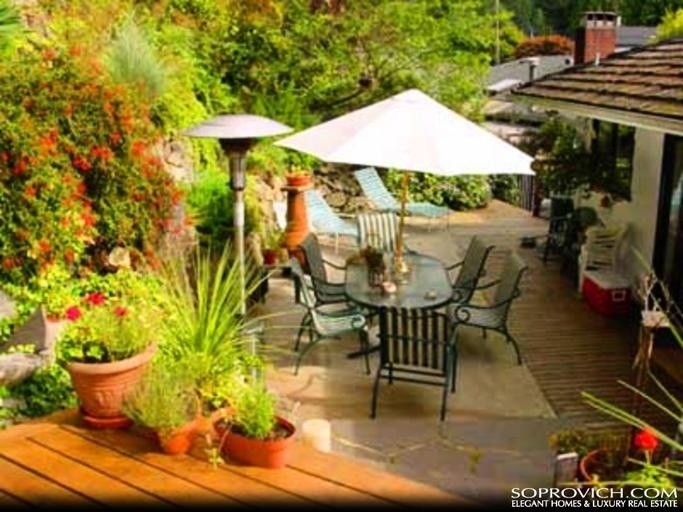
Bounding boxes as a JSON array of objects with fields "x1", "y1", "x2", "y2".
[{"x1": 58, "y1": 347, "x2": 157, "y2": 429}]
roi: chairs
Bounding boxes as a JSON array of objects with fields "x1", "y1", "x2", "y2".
[
  {"x1": 273, "y1": 166, "x2": 528, "y2": 422},
  {"x1": 541, "y1": 205, "x2": 629, "y2": 293}
]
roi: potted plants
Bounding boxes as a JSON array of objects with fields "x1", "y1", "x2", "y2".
[{"x1": 126, "y1": 233, "x2": 297, "y2": 471}]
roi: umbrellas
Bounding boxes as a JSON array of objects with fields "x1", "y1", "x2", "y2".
[{"x1": 271, "y1": 86, "x2": 538, "y2": 272}]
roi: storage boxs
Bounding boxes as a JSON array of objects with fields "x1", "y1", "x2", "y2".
[{"x1": 583, "y1": 268, "x2": 632, "y2": 317}]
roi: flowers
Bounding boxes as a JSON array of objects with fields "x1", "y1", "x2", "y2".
[{"x1": 56, "y1": 288, "x2": 152, "y2": 362}]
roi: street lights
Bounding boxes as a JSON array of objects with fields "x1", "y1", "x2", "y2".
[{"x1": 181, "y1": 113, "x2": 295, "y2": 379}]
